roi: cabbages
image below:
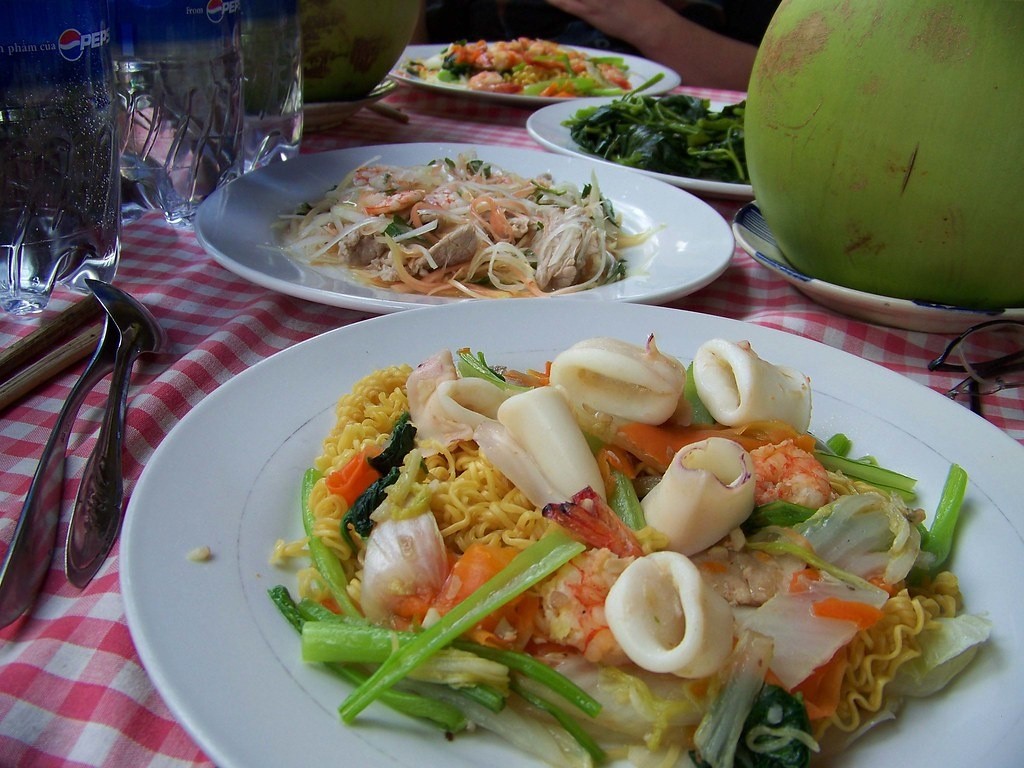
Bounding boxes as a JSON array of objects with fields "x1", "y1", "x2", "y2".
[{"x1": 381, "y1": 489, "x2": 992, "y2": 767}]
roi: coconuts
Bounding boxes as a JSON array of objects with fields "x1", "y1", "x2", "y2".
[
  {"x1": 745, "y1": 0, "x2": 1024, "y2": 315},
  {"x1": 238, "y1": 0, "x2": 429, "y2": 114}
]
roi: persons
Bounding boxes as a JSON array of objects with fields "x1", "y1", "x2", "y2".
[{"x1": 410, "y1": 0, "x2": 780, "y2": 91}]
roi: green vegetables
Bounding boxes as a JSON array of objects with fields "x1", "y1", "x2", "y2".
[
  {"x1": 292, "y1": 156, "x2": 626, "y2": 290},
  {"x1": 271, "y1": 344, "x2": 968, "y2": 768},
  {"x1": 561, "y1": 72, "x2": 749, "y2": 189},
  {"x1": 430, "y1": 37, "x2": 629, "y2": 96}
]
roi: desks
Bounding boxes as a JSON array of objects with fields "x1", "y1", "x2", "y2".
[{"x1": 0, "y1": 82, "x2": 1024, "y2": 768}]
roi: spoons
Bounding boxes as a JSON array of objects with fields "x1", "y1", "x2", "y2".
[{"x1": 0, "y1": 278, "x2": 166, "y2": 629}]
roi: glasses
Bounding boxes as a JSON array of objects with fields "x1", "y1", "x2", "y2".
[{"x1": 927, "y1": 318, "x2": 1024, "y2": 417}]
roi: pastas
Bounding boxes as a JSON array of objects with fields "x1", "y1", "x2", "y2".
[{"x1": 270, "y1": 361, "x2": 961, "y2": 768}]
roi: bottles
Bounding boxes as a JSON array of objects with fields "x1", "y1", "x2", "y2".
[
  {"x1": 118, "y1": 0, "x2": 248, "y2": 222},
  {"x1": 0, "y1": 0, "x2": 122, "y2": 316},
  {"x1": 237, "y1": 0, "x2": 306, "y2": 172}
]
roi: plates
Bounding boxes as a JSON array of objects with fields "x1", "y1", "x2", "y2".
[
  {"x1": 731, "y1": 200, "x2": 1023, "y2": 334},
  {"x1": 525, "y1": 95, "x2": 753, "y2": 202},
  {"x1": 195, "y1": 142, "x2": 734, "y2": 315},
  {"x1": 117, "y1": 295, "x2": 1024, "y2": 768},
  {"x1": 297, "y1": 83, "x2": 401, "y2": 135},
  {"x1": 388, "y1": 43, "x2": 681, "y2": 106}
]
